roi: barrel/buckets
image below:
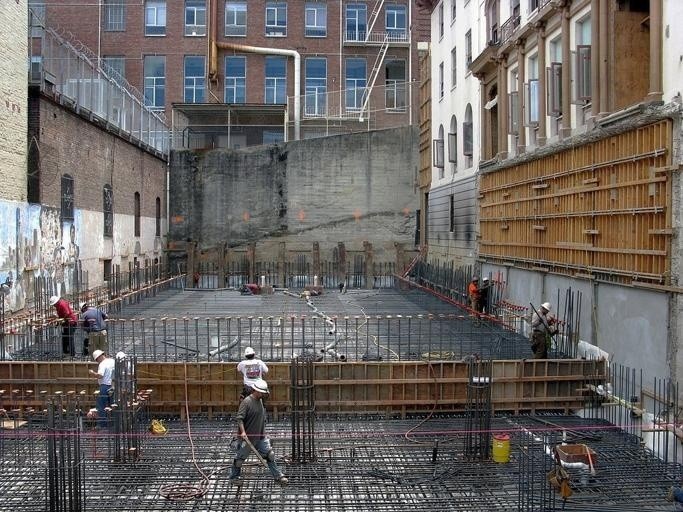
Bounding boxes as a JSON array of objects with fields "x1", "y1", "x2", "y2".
[{"x1": 492, "y1": 435, "x2": 510, "y2": 463}]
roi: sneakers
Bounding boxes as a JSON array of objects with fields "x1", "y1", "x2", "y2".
[
  {"x1": 229, "y1": 478, "x2": 242, "y2": 486},
  {"x1": 279, "y1": 477, "x2": 289, "y2": 484}
]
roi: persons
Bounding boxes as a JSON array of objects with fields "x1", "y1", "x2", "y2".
[
  {"x1": 469, "y1": 277, "x2": 480, "y2": 321},
  {"x1": 80, "y1": 302, "x2": 108, "y2": 356},
  {"x1": 529, "y1": 302, "x2": 552, "y2": 358},
  {"x1": 548, "y1": 312, "x2": 554, "y2": 325},
  {"x1": 229, "y1": 380, "x2": 287, "y2": 486},
  {"x1": 106, "y1": 351, "x2": 136, "y2": 396},
  {"x1": 89, "y1": 349, "x2": 116, "y2": 429},
  {"x1": 480, "y1": 276, "x2": 492, "y2": 311},
  {"x1": 236, "y1": 346, "x2": 269, "y2": 402},
  {"x1": 242, "y1": 283, "x2": 259, "y2": 295},
  {"x1": 193, "y1": 271, "x2": 200, "y2": 288},
  {"x1": 48, "y1": 295, "x2": 77, "y2": 353}
]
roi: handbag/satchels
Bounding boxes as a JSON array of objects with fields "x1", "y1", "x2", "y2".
[{"x1": 150, "y1": 420, "x2": 167, "y2": 434}]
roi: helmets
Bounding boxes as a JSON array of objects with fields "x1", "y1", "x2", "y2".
[
  {"x1": 471, "y1": 277, "x2": 489, "y2": 282},
  {"x1": 116, "y1": 351, "x2": 127, "y2": 362},
  {"x1": 79, "y1": 302, "x2": 87, "y2": 311},
  {"x1": 49, "y1": 296, "x2": 62, "y2": 306},
  {"x1": 252, "y1": 380, "x2": 270, "y2": 394},
  {"x1": 93, "y1": 350, "x2": 105, "y2": 361},
  {"x1": 541, "y1": 302, "x2": 552, "y2": 312},
  {"x1": 244, "y1": 347, "x2": 255, "y2": 356}
]
